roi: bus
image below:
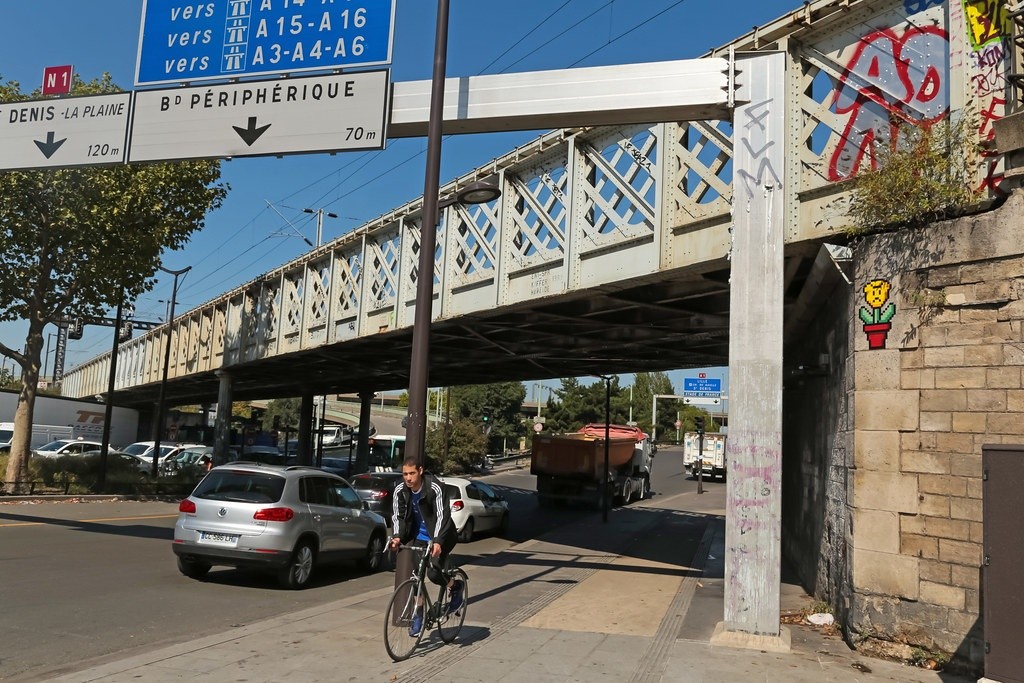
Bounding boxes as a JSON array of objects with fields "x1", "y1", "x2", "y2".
[{"x1": 369, "y1": 434, "x2": 407, "y2": 472}]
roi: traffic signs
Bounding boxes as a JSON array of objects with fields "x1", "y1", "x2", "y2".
[
  {"x1": 0, "y1": 91, "x2": 136, "y2": 168},
  {"x1": 126, "y1": 63, "x2": 390, "y2": 159}
]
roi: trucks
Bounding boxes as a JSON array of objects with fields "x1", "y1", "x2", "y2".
[
  {"x1": 323, "y1": 425, "x2": 351, "y2": 446},
  {"x1": 0, "y1": 421, "x2": 74, "y2": 451},
  {"x1": 683, "y1": 429, "x2": 727, "y2": 483}
]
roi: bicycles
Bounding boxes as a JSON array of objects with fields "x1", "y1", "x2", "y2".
[{"x1": 381, "y1": 536, "x2": 470, "y2": 665}]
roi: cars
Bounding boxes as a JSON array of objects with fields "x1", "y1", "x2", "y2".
[
  {"x1": 1, "y1": 444, "x2": 45, "y2": 465},
  {"x1": 349, "y1": 472, "x2": 405, "y2": 526},
  {"x1": 119, "y1": 439, "x2": 299, "y2": 477},
  {"x1": 34, "y1": 437, "x2": 115, "y2": 460},
  {"x1": 436, "y1": 478, "x2": 510, "y2": 541}
]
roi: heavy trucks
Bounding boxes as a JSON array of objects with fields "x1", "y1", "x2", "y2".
[{"x1": 529, "y1": 421, "x2": 658, "y2": 508}]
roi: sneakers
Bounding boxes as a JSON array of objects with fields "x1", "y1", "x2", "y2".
[
  {"x1": 409, "y1": 614, "x2": 423, "y2": 637},
  {"x1": 450, "y1": 579, "x2": 465, "y2": 614}
]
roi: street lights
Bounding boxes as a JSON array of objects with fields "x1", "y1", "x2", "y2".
[{"x1": 402, "y1": 180, "x2": 504, "y2": 469}]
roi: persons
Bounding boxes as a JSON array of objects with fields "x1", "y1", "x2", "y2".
[{"x1": 389, "y1": 456, "x2": 464, "y2": 637}]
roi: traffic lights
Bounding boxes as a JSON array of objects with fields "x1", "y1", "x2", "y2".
[{"x1": 483, "y1": 405, "x2": 490, "y2": 423}]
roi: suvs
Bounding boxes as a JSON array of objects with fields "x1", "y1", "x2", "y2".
[{"x1": 173, "y1": 460, "x2": 388, "y2": 590}]
